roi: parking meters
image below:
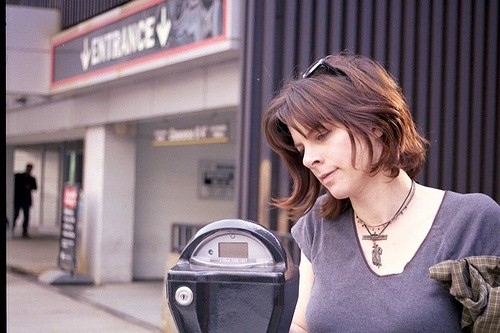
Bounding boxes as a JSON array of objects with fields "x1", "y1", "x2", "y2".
[{"x1": 164, "y1": 217, "x2": 301, "y2": 332}]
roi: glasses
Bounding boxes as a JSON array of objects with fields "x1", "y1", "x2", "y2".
[{"x1": 303, "y1": 55, "x2": 350, "y2": 81}]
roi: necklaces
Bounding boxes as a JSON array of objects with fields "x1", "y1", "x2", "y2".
[{"x1": 355, "y1": 178, "x2": 415, "y2": 267}]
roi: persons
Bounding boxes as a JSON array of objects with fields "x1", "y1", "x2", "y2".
[
  {"x1": 13, "y1": 163, "x2": 40, "y2": 238},
  {"x1": 259, "y1": 48, "x2": 500, "y2": 331}
]
